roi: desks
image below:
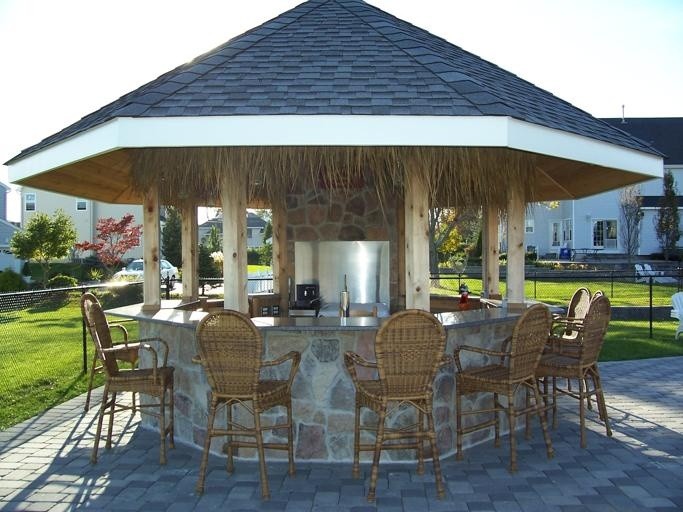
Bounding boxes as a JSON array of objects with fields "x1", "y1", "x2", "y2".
[{"x1": 210, "y1": 290, "x2": 278, "y2": 317}]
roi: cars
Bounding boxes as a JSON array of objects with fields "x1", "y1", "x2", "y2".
[{"x1": 111, "y1": 258, "x2": 179, "y2": 289}]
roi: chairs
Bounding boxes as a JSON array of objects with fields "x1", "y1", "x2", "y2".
[
  {"x1": 343, "y1": 309, "x2": 446, "y2": 503},
  {"x1": 81, "y1": 292, "x2": 175, "y2": 465},
  {"x1": 293, "y1": 283, "x2": 320, "y2": 317},
  {"x1": 189, "y1": 309, "x2": 301, "y2": 500},
  {"x1": 454, "y1": 303, "x2": 555, "y2": 473},
  {"x1": 526, "y1": 287, "x2": 611, "y2": 449}
]
